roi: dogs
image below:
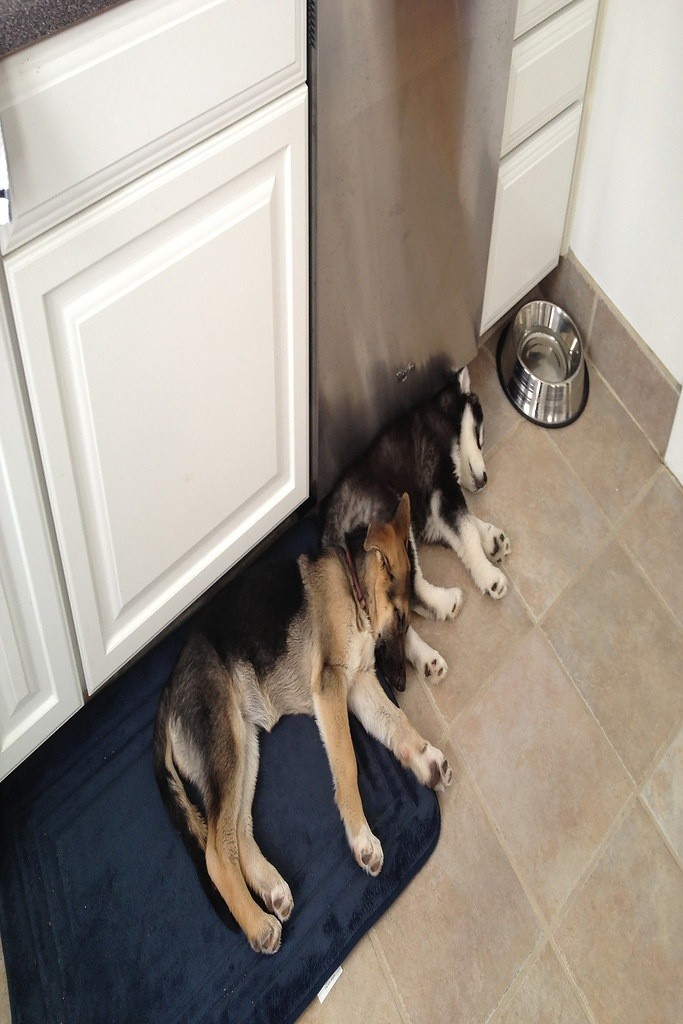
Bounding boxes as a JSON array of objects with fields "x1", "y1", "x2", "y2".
[
  {"x1": 152, "y1": 490, "x2": 454, "y2": 956},
  {"x1": 322, "y1": 364, "x2": 515, "y2": 689}
]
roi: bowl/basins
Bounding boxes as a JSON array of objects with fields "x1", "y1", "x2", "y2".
[{"x1": 497, "y1": 300, "x2": 590, "y2": 429}]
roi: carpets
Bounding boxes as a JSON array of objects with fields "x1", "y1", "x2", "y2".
[{"x1": 1, "y1": 559, "x2": 441, "y2": 1024}]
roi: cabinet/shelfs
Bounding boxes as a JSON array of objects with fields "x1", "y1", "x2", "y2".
[{"x1": 0, "y1": 0, "x2": 599, "y2": 789}]
